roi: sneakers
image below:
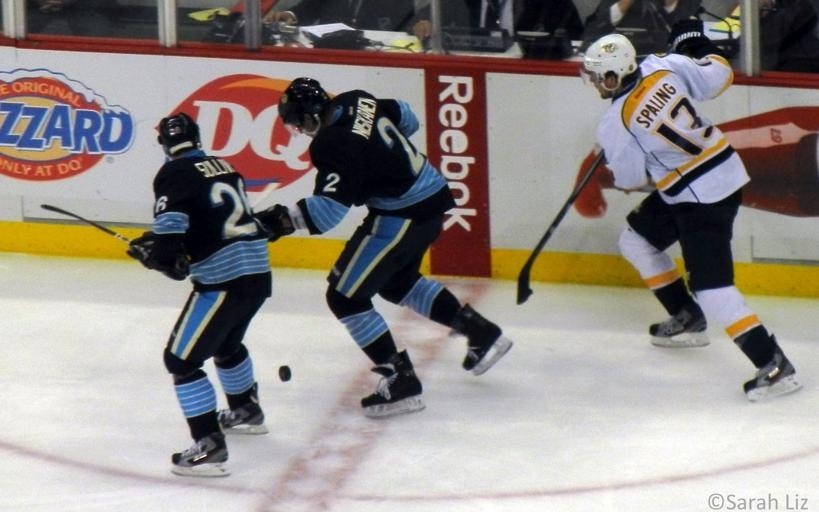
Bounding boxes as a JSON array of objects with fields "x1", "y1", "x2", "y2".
[
  {"x1": 216, "y1": 401, "x2": 264, "y2": 429},
  {"x1": 171, "y1": 431, "x2": 228, "y2": 466},
  {"x1": 648, "y1": 298, "x2": 706, "y2": 338},
  {"x1": 744, "y1": 343, "x2": 796, "y2": 391}
]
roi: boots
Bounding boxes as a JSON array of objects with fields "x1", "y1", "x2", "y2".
[
  {"x1": 360, "y1": 348, "x2": 422, "y2": 406},
  {"x1": 449, "y1": 303, "x2": 501, "y2": 370}
]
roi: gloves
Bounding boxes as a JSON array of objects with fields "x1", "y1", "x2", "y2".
[
  {"x1": 127, "y1": 234, "x2": 175, "y2": 273},
  {"x1": 667, "y1": 19, "x2": 727, "y2": 60},
  {"x1": 251, "y1": 204, "x2": 296, "y2": 243}
]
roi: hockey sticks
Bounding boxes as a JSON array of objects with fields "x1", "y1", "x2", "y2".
[
  {"x1": 517, "y1": 152, "x2": 606, "y2": 302},
  {"x1": 40, "y1": 204, "x2": 146, "y2": 249}
]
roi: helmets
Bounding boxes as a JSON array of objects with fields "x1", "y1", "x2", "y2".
[
  {"x1": 278, "y1": 77, "x2": 330, "y2": 138},
  {"x1": 157, "y1": 113, "x2": 201, "y2": 159},
  {"x1": 580, "y1": 32, "x2": 638, "y2": 94}
]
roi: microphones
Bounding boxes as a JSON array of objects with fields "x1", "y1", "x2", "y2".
[{"x1": 697, "y1": 6, "x2": 739, "y2": 58}]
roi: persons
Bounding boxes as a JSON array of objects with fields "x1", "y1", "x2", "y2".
[
  {"x1": 254, "y1": 74, "x2": 502, "y2": 407},
  {"x1": 125, "y1": 110, "x2": 276, "y2": 467},
  {"x1": 176, "y1": 0, "x2": 708, "y2": 65},
  {"x1": 578, "y1": 34, "x2": 798, "y2": 391}
]
